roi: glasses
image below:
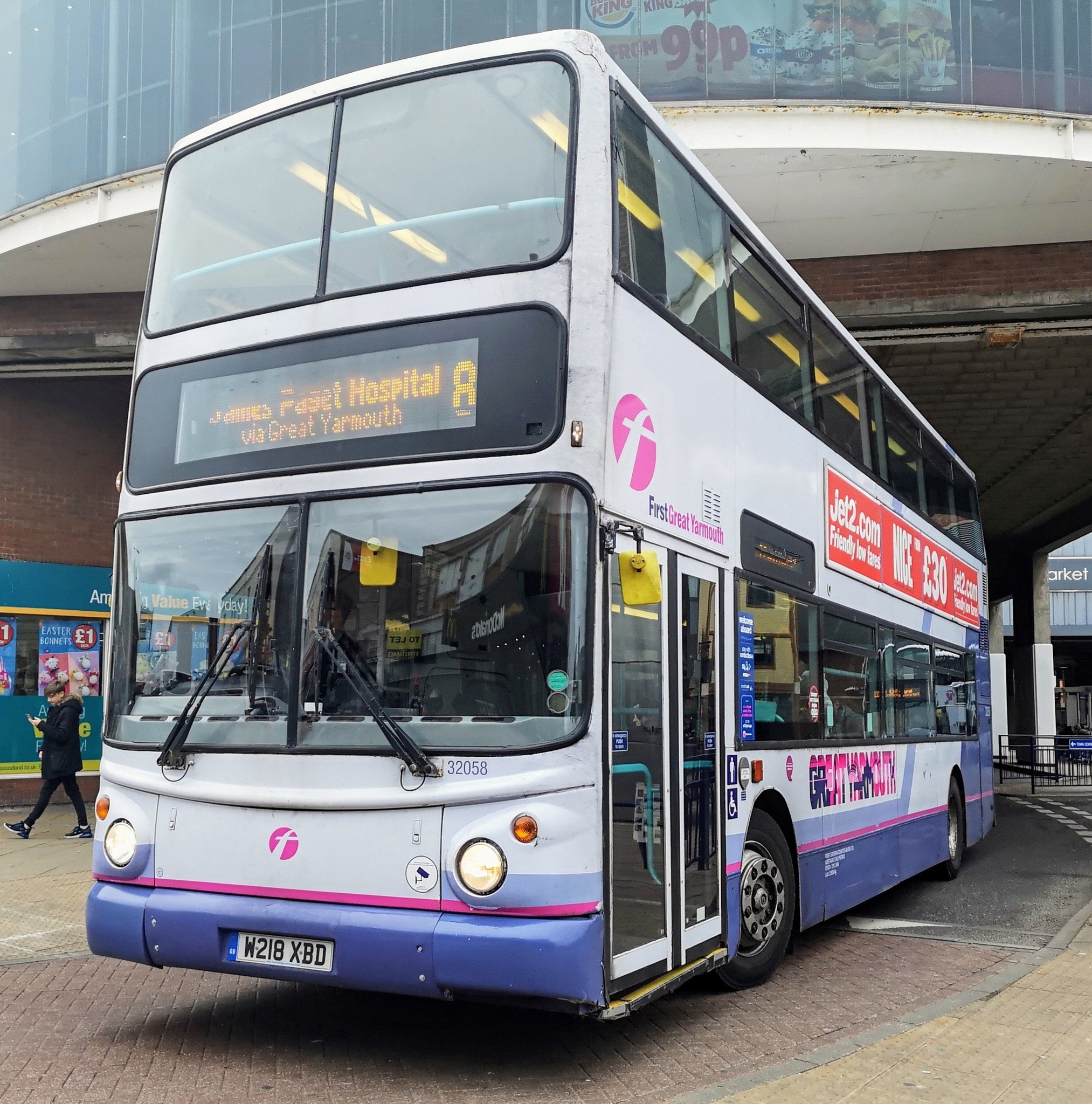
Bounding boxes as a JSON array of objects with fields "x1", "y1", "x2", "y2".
[{"x1": 47, "y1": 693, "x2": 56, "y2": 697}]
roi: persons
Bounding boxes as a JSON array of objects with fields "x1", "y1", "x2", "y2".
[
  {"x1": 687, "y1": 670, "x2": 716, "y2": 730},
  {"x1": 625, "y1": 671, "x2": 663, "y2": 742},
  {"x1": 449, "y1": 579, "x2": 562, "y2": 693},
  {"x1": 3, "y1": 680, "x2": 93, "y2": 857},
  {"x1": 831, "y1": 698, "x2": 865, "y2": 739},
  {"x1": 258, "y1": 588, "x2": 371, "y2": 714}
]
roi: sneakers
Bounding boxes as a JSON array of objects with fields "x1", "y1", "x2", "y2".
[
  {"x1": 4, "y1": 821, "x2": 32, "y2": 838},
  {"x1": 65, "y1": 825, "x2": 92, "y2": 838}
]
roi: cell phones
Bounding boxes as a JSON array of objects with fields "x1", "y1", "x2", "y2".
[{"x1": 24, "y1": 714, "x2": 34, "y2": 721}]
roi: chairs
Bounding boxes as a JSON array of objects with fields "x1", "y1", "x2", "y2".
[
  {"x1": 746, "y1": 367, "x2": 761, "y2": 382},
  {"x1": 652, "y1": 291, "x2": 671, "y2": 310},
  {"x1": 840, "y1": 443, "x2": 852, "y2": 454},
  {"x1": 818, "y1": 421, "x2": 826, "y2": 435},
  {"x1": 929, "y1": 513, "x2": 970, "y2": 549},
  {"x1": 782, "y1": 396, "x2": 796, "y2": 411},
  {"x1": 907, "y1": 727, "x2": 931, "y2": 737},
  {"x1": 903, "y1": 493, "x2": 911, "y2": 503}
]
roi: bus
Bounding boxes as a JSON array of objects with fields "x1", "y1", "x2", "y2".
[
  {"x1": 862, "y1": 642, "x2": 976, "y2": 736},
  {"x1": 85, "y1": 28, "x2": 996, "y2": 1024}
]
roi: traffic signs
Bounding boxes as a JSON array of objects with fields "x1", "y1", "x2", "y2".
[{"x1": 1069, "y1": 739, "x2": 1092, "y2": 750}]
